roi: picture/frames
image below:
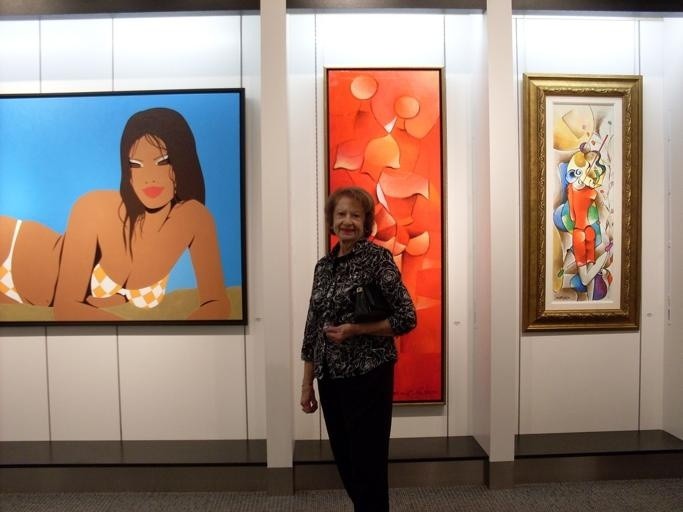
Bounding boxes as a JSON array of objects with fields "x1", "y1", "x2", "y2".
[
  {"x1": 520, "y1": 72, "x2": 643, "y2": 335},
  {"x1": 0, "y1": 88, "x2": 253, "y2": 329},
  {"x1": 321, "y1": 66, "x2": 450, "y2": 407}
]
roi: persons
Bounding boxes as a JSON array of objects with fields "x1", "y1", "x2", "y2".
[
  {"x1": 566, "y1": 143, "x2": 614, "y2": 303},
  {"x1": 0, "y1": 109, "x2": 231, "y2": 322},
  {"x1": 301, "y1": 188, "x2": 416, "y2": 511}
]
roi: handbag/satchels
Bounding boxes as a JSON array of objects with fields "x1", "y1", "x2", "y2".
[{"x1": 352, "y1": 284, "x2": 395, "y2": 322}]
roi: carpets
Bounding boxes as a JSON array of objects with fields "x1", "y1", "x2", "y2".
[{"x1": 0, "y1": 479, "x2": 683, "y2": 512}]
